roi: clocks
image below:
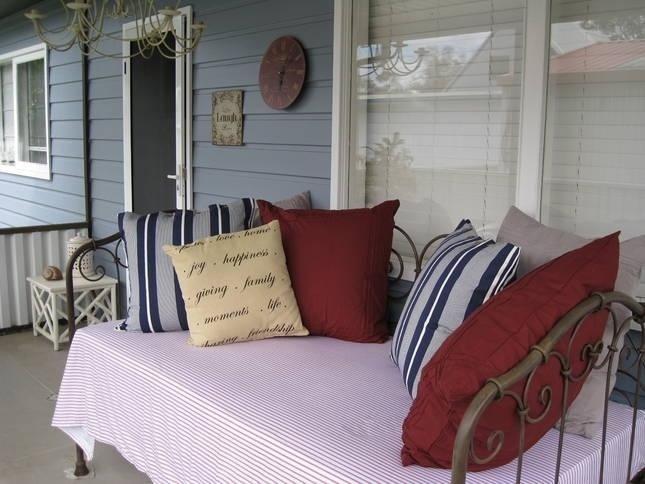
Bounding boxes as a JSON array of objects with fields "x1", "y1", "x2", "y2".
[{"x1": 257, "y1": 36, "x2": 308, "y2": 110}]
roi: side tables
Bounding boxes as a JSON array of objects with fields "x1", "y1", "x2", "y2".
[{"x1": 26, "y1": 270, "x2": 120, "y2": 351}]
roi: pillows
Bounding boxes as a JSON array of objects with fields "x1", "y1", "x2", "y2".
[
  {"x1": 116, "y1": 190, "x2": 399, "y2": 346},
  {"x1": 390, "y1": 207, "x2": 645, "y2": 470}
]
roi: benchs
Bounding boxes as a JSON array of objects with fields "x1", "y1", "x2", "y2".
[{"x1": 64, "y1": 195, "x2": 643, "y2": 483}]
